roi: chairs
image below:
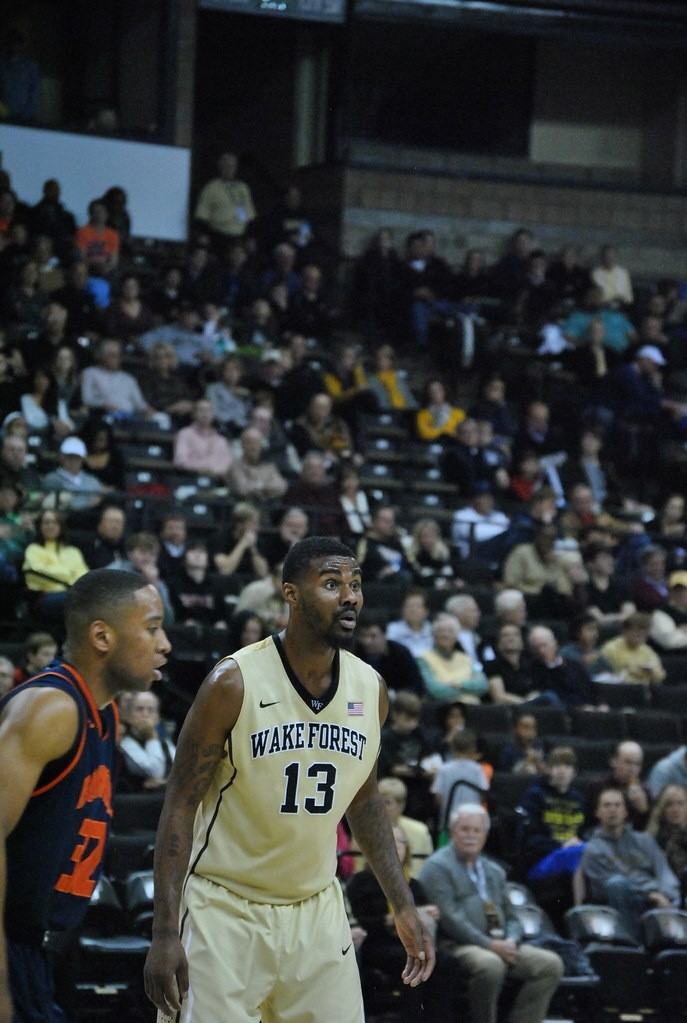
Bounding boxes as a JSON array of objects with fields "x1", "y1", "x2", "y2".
[{"x1": 0, "y1": 236, "x2": 686, "y2": 1023}]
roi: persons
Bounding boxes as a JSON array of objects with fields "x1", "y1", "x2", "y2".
[
  {"x1": 1, "y1": 111, "x2": 687, "y2": 1023},
  {"x1": 143, "y1": 536, "x2": 436, "y2": 1023},
  {"x1": 194, "y1": 152, "x2": 257, "y2": 243},
  {"x1": 0, "y1": 570, "x2": 171, "y2": 1023},
  {"x1": 419, "y1": 802, "x2": 565, "y2": 1023},
  {"x1": 582, "y1": 788, "x2": 681, "y2": 917}
]
